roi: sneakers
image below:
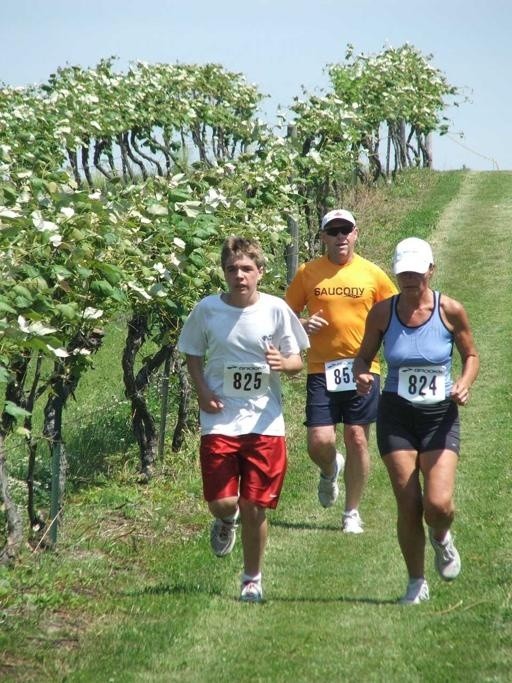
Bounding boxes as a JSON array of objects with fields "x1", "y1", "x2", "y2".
[
  {"x1": 427, "y1": 525, "x2": 462, "y2": 581},
  {"x1": 312, "y1": 452, "x2": 346, "y2": 508},
  {"x1": 209, "y1": 502, "x2": 239, "y2": 557},
  {"x1": 239, "y1": 571, "x2": 264, "y2": 602},
  {"x1": 340, "y1": 506, "x2": 364, "y2": 534},
  {"x1": 399, "y1": 578, "x2": 430, "y2": 604}
]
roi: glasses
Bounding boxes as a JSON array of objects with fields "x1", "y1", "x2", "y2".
[{"x1": 324, "y1": 225, "x2": 355, "y2": 235}]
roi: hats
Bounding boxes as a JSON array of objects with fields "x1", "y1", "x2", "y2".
[
  {"x1": 321, "y1": 208, "x2": 356, "y2": 235},
  {"x1": 391, "y1": 237, "x2": 434, "y2": 275}
]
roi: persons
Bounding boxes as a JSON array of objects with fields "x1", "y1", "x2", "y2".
[
  {"x1": 352, "y1": 237, "x2": 480, "y2": 603},
  {"x1": 286, "y1": 209, "x2": 400, "y2": 534},
  {"x1": 178, "y1": 237, "x2": 311, "y2": 602}
]
